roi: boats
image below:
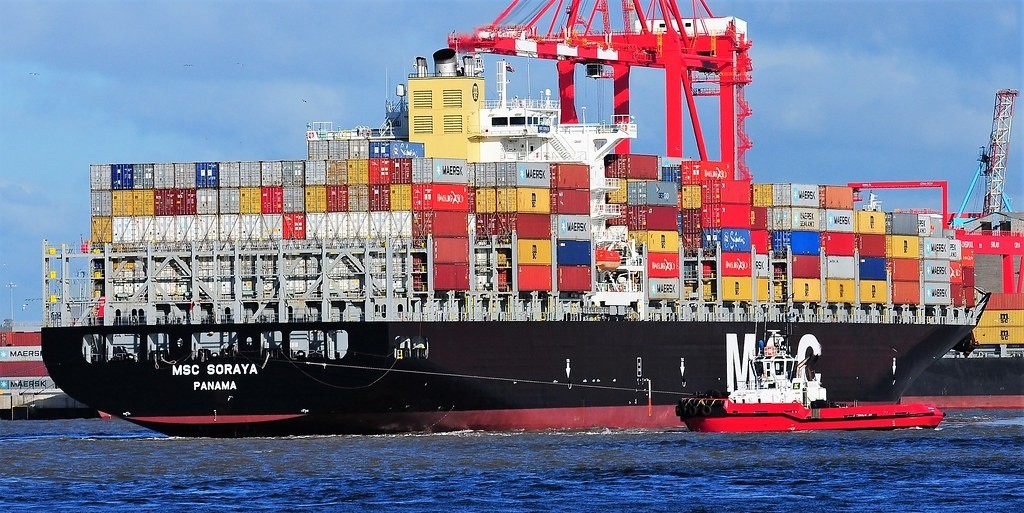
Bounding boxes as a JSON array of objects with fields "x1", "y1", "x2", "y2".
[{"x1": 675, "y1": 332, "x2": 947, "y2": 434}]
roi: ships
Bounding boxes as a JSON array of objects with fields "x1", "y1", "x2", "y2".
[{"x1": 40, "y1": 46, "x2": 990, "y2": 438}]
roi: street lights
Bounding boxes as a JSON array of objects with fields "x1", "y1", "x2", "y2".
[{"x1": 6, "y1": 282, "x2": 17, "y2": 321}]
